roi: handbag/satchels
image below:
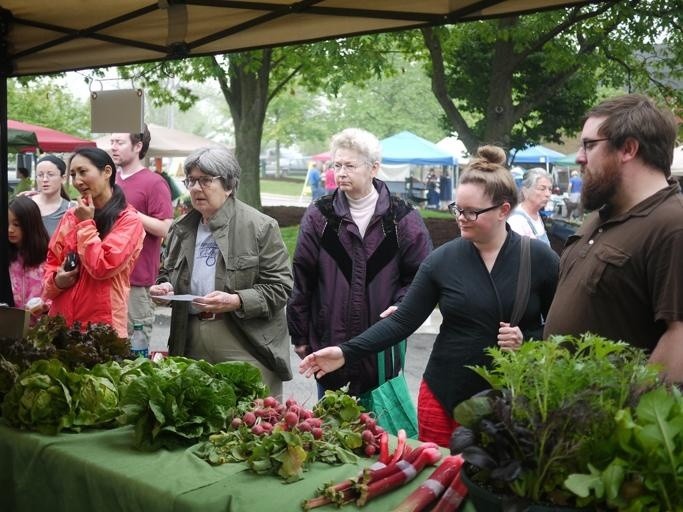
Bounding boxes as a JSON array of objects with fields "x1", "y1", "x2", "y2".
[{"x1": 362, "y1": 372, "x2": 419, "y2": 441}]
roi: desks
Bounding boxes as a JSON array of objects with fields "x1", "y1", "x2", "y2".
[{"x1": 0, "y1": 420, "x2": 476, "y2": 511}]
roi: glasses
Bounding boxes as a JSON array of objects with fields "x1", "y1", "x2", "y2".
[
  {"x1": 447, "y1": 201, "x2": 506, "y2": 222},
  {"x1": 581, "y1": 137, "x2": 608, "y2": 155},
  {"x1": 182, "y1": 175, "x2": 221, "y2": 188},
  {"x1": 334, "y1": 158, "x2": 367, "y2": 173}
]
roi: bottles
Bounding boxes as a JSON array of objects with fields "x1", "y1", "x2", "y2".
[{"x1": 128, "y1": 325, "x2": 150, "y2": 359}]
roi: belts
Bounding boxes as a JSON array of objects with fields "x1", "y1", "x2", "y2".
[{"x1": 195, "y1": 311, "x2": 225, "y2": 321}]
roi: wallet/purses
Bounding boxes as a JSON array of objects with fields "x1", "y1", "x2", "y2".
[{"x1": 65, "y1": 253, "x2": 77, "y2": 271}]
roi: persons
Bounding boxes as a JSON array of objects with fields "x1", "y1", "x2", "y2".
[
  {"x1": 286, "y1": 127, "x2": 435, "y2": 414},
  {"x1": 306, "y1": 160, "x2": 324, "y2": 201},
  {"x1": 424, "y1": 169, "x2": 440, "y2": 210},
  {"x1": 302, "y1": 144, "x2": 562, "y2": 448},
  {"x1": 152, "y1": 147, "x2": 295, "y2": 405},
  {"x1": 14, "y1": 167, "x2": 33, "y2": 192},
  {"x1": 544, "y1": 91, "x2": 682, "y2": 395},
  {"x1": 27, "y1": 154, "x2": 76, "y2": 234},
  {"x1": 8, "y1": 194, "x2": 55, "y2": 323},
  {"x1": 39, "y1": 147, "x2": 148, "y2": 340},
  {"x1": 509, "y1": 167, "x2": 556, "y2": 250},
  {"x1": 567, "y1": 168, "x2": 584, "y2": 221},
  {"x1": 326, "y1": 161, "x2": 339, "y2": 194},
  {"x1": 105, "y1": 121, "x2": 174, "y2": 339}
]
